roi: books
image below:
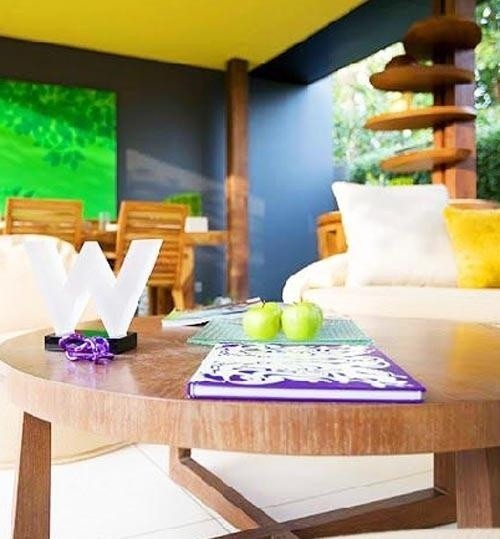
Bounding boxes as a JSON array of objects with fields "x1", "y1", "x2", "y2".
[
  {"x1": 186, "y1": 342, "x2": 426, "y2": 403},
  {"x1": 161, "y1": 296, "x2": 263, "y2": 328}
]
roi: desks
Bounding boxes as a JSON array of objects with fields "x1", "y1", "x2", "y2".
[{"x1": 1, "y1": 318, "x2": 500, "y2": 537}]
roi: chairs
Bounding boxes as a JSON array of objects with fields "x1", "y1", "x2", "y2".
[
  {"x1": 283, "y1": 199, "x2": 500, "y2": 321},
  {"x1": 1, "y1": 196, "x2": 190, "y2": 313}
]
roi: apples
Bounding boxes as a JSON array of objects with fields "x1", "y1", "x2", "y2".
[
  {"x1": 280, "y1": 301, "x2": 324, "y2": 342},
  {"x1": 241, "y1": 300, "x2": 283, "y2": 341}
]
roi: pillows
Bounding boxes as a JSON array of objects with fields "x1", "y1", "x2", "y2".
[{"x1": 331, "y1": 181, "x2": 499, "y2": 288}]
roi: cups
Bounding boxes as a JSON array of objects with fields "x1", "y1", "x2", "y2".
[{"x1": 99, "y1": 212, "x2": 110, "y2": 231}]
